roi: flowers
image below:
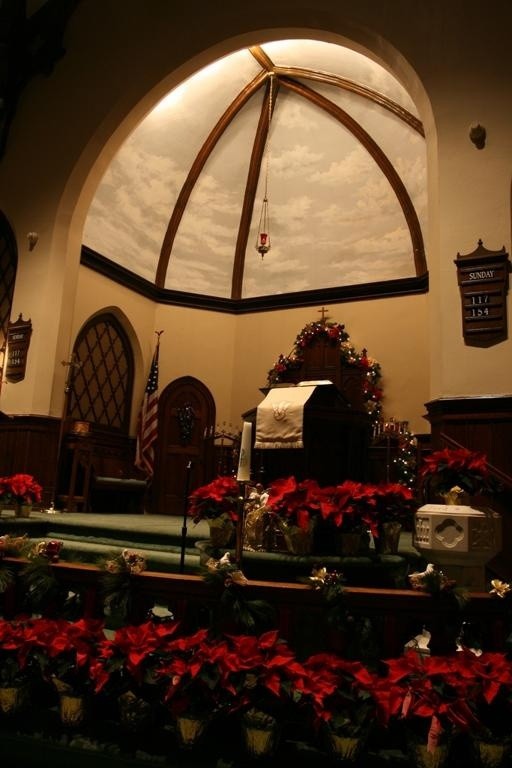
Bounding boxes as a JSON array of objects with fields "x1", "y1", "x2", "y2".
[
  {"x1": 2, "y1": 320, "x2": 510, "y2": 550},
  {"x1": 0, "y1": 531, "x2": 512, "y2": 754}
]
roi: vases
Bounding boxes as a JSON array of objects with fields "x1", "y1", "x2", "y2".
[
  {"x1": 415, "y1": 743, "x2": 447, "y2": 768},
  {"x1": 479, "y1": 742, "x2": 504, "y2": 768},
  {"x1": 331, "y1": 733, "x2": 358, "y2": 761}
]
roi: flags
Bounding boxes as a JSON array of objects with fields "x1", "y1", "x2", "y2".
[{"x1": 135, "y1": 343, "x2": 159, "y2": 487}]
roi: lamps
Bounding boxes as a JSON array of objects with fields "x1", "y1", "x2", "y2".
[{"x1": 255, "y1": 71, "x2": 277, "y2": 260}]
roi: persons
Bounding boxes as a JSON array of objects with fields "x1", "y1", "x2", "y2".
[{"x1": 242, "y1": 482, "x2": 270, "y2": 553}]
